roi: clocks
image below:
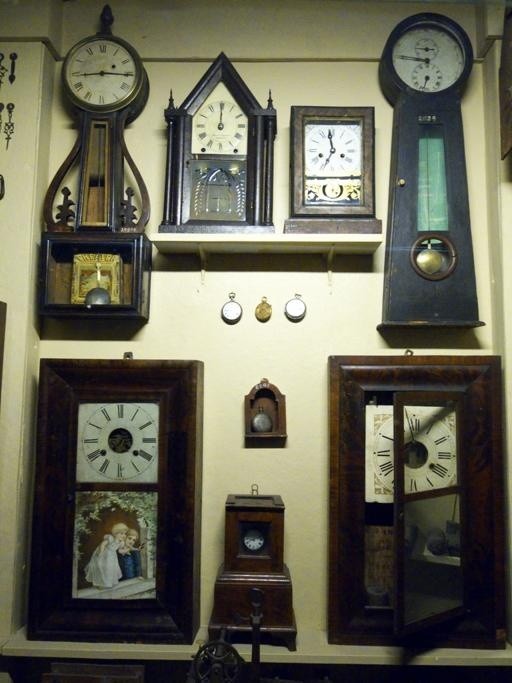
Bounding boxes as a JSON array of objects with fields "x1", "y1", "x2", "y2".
[
  {"x1": 327, "y1": 351, "x2": 506, "y2": 650},
  {"x1": 37, "y1": 5, "x2": 152, "y2": 340},
  {"x1": 208, "y1": 484, "x2": 297, "y2": 651},
  {"x1": 27, "y1": 352, "x2": 204, "y2": 645},
  {"x1": 158, "y1": 52, "x2": 277, "y2": 234},
  {"x1": 283, "y1": 106, "x2": 382, "y2": 234},
  {"x1": 377, "y1": 12, "x2": 486, "y2": 330}
]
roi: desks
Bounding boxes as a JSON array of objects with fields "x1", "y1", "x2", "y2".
[{"x1": 0, "y1": 629, "x2": 512, "y2": 683}]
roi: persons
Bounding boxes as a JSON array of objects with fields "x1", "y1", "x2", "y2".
[
  {"x1": 84, "y1": 523, "x2": 142, "y2": 590},
  {"x1": 115, "y1": 528, "x2": 144, "y2": 582}
]
